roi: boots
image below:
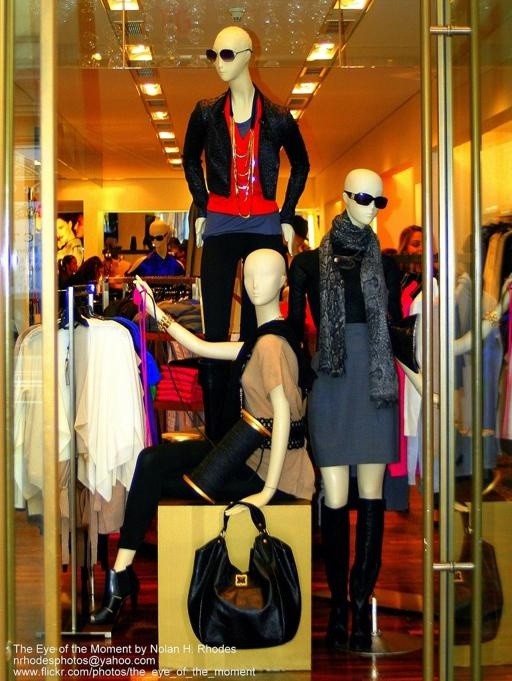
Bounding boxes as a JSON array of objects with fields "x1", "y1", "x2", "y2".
[
  {"x1": 348, "y1": 495, "x2": 388, "y2": 653},
  {"x1": 320, "y1": 499, "x2": 351, "y2": 656}
]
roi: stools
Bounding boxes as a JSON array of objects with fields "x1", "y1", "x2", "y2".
[{"x1": 157, "y1": 497, "x2": 314, "y2": 677}]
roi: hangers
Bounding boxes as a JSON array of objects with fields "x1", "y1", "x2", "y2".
[
  {"x1": 21, "y1": 285, "x2": 109, "y2": 347},
  {"x1": 119, "y1": 273, "x2": 194, "y2": 305}
]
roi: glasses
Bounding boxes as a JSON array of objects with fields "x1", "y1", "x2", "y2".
[
  {"x1": 149, "y1": 231, "x2": 169, "y2": 241},
  {"x1": 205, "y1": 47, "x2": 254, "y2": 63},
  {"x1": 343, "y1": 189, "x2": 389, "y2": 209}
]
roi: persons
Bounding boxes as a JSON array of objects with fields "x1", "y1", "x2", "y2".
[
  {"x1": 99, "y1": 248, "x2": 119, "y2": 277},
  {"x1": 104, "y1": 237, "x2": 121, "y2": 258},
  {"x1": 127, "y1": 221, "x2": 185, "y2": 275},
  {"x1": 454, "y1": 285, "x2": 512, "y2": 452},
  {"x1": 283, "y1": 167, "x2": 440, "y2": 652},
  {"x1": 397, "y1": 226, "x2": 436, "y2": 277},
  {"x1": 89, "y1": 248, "x2": 314, "y2": 622},
  {"x1": 183, "y1": 27, "x2": 310, "y2": 432},
  {"x1": 67, "y1": 256, "x2": 103, "y2": 304},
  {"x1": 55, "y1": 213, "x2": 84, "y2": 275},
  {"x1": 57, "y1": 254, "x2": 78, "y2": 308}
]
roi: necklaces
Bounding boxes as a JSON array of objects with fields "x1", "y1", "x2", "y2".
[
  {"x1": 104, "y1": 259, "x2": 113, "y2": 274},
  {"x1": 228, "y1": 94, "x2": 257, "y2": 199}
]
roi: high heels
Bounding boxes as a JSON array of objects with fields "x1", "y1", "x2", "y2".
[{"x1": 88, "y1": 561, "x2": 141, "y2": 626}]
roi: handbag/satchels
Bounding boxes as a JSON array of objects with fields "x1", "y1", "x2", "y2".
[
  {"x1": 185, "y1": 500, "x2": 304, "y2": 650},
  {"x1": 452, "y1": 498, "x2": 504, "y2": 647}
]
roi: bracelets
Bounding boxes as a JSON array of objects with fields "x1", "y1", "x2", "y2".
[{"x1": 156, "y1": 313, "x2": 174, "y2": 331}]
